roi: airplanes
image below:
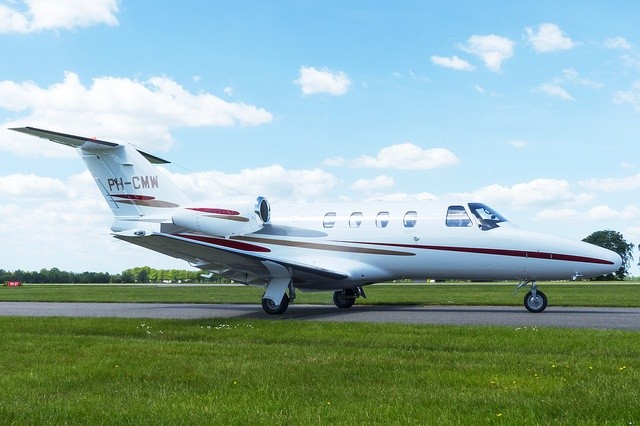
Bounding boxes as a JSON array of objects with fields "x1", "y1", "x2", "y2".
[{"x1": 7, "y1": 121, "x2": 623, "y2": 316}]
